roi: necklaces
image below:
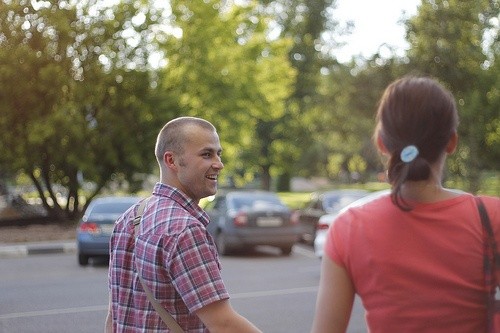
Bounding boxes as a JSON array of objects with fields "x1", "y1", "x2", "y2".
[{"x1": 400, "y1": 181, "x2": 440, "y2": 190}]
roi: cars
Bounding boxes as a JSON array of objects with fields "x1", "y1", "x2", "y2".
[
  {"x1": 289, "y1": 188, "x2": 371, "y2": 242},
  {"x1": 314, "y1": 213, "x2": 338, "y2": 262},
  {"x1": 204, "y1": 190, "x2": 302, "y2": 255},
  {"x1": 77, "y1": 195, "x2": 143, "y2": 265}
]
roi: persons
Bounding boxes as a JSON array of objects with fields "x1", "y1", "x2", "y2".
[
  {"x1": 105, "y1": 116, "x2": 264, "y2": 333},
  {"x1": 312, "y1": 75, "x2": 500, "y2": 333}
]
